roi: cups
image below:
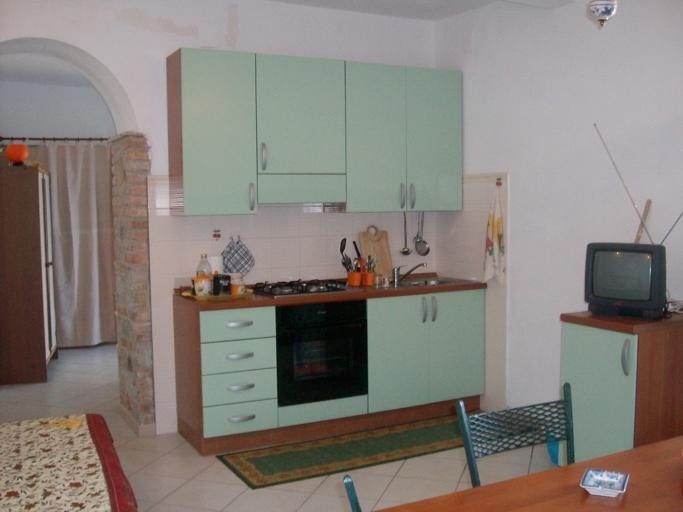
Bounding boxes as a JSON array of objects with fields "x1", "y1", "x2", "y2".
[
  {"x1": 361, "y1": 272, "x2": 375, "y2": 288},
  {"x1": 348, "y1": 271, "x2": 361, "y2": 288}
]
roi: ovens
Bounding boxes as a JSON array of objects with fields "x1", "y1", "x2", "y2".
[{"x1": 275, "y1": 299, "x2": 369, "y2": 407}]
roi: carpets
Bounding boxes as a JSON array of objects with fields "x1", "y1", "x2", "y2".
[{"x1": 218, "y1": 410, "x2": 544, "y2": 490}]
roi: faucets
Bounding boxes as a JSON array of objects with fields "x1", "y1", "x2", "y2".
[{"x1": 391, "y1": 262, "x2": 427, "y2": 289}]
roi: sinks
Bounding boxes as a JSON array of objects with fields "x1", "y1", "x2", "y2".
[{"x1": 391, "y1": 276, "x2": 466, "y2": 287}]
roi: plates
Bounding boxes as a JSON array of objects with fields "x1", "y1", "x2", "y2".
[
  {"x1": 579, "y1": 467, "x2": 630, "y2": 498},
  {"x1": 181, "y1": 289, "x2": 253, "y2": 302}
]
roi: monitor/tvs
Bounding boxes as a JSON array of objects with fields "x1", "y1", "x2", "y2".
[{"x1": 584, "y1": 241, "x2": 667, "y2": 322}]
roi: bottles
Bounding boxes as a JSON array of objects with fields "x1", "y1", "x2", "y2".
[{"x1": 196, "y1": 253, "x2": 213, "y2": 296}]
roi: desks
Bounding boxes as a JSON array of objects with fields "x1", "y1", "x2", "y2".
[{"x1": 369, "y1": 437, "x2": 683, "y2": 512}]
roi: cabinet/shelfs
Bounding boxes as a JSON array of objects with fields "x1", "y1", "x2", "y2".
[
  {"x1": 347, "y1": 60, "x2": 465, "y2": 212},
  {"x1": 1, "y1": 166, "x2": 58, "y2": 385},
  {"x1": 369, "y1": 286, "x2": 487, "y2": 434},
  {"x1": 167, "y1": 48, "x2": 346, "y2": 217},
  {"x1": 555, "y1": 313, "x2": 683, "y2": 464},
  {"x1": 173, "y1": 297, "x2": 277, "y2": 456}
]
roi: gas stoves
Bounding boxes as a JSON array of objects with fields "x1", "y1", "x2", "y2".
[{"x1": 256, "y1": 280, "x2": 346, "y2": 295}]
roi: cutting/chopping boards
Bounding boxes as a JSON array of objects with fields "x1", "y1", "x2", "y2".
[{"x1": 358, "y1": 224, "x2": 393, "y2": 284}]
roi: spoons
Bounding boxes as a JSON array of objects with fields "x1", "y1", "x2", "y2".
[
  {"x1": 400, "y1": 211, "x2": 413, "y2": 256},
  {"x1": 415, "y1": 211, "x2": 430, "y2": 256}
]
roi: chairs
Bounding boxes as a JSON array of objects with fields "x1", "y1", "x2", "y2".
[{"x1": 456, "y1": 382, "x2": 580, "y2": 486}]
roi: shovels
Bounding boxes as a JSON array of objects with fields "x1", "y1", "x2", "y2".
[{"x1": 340, "y1": 238, "x2": 346, "y2": 263}]
